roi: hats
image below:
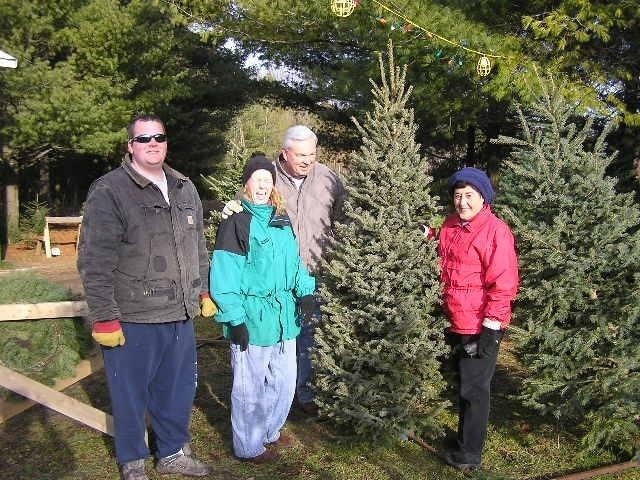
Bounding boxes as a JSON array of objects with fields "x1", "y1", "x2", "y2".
[
  {"x1": 448, "y1": 168, "x2": 494, "y2": 203},
  {"x1": 242, "y1": 151, "x2": 276, "y2": 186}
]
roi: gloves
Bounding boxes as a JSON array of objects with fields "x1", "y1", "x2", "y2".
[
  {"x1": 92, "y1": 319, "x2": 126, "y2": 348},
  {"x1": 302, "y1": 295, "x2": 316, "y2": 312},
  {"x1": 200, "y1": 293, "x2": 219, "y2": 317},
  {"x1": 479, "y1": 326, "x2": 496, "y2": 359},
  {"x1": 231, "y1": 323, "x2": 249, "y2": 350}
]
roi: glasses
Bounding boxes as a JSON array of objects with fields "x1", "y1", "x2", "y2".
[{"x1": 131, "y1": 134, "x2": 167, "y2": 145}]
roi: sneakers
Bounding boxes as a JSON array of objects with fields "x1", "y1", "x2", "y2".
[
  {"x1": 120, "y1": 458, "x2": 149, "y2": 480},
  {"x1": 236, "y1": 447, "x2": 280, "y2": 463},
  {"x1": 265, "y1": 434, "x2": 294, "y2": 448},
  {"x1": 443, "y1": 432, "x2": 485, "y2": 468},
  {"x1": 156, "y1": 448, "x2": 213, "y2": 476},
  {"x1": 287, "y1": 401, "x2": 318, "y2": 420}
]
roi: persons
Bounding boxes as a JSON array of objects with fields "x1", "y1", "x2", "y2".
[
  {"x1": 207, "y1": 150, "x2": 319, "y2": 466},
  {"x1": 415, "y1": 166, "x2": 521, "y2": 467},
  {"x1": 219, "y1": 123, "x2": 357, "y2": 423},
  {"x1": 73, "y1": 113, "x2": 217, "y2": 480}
]
